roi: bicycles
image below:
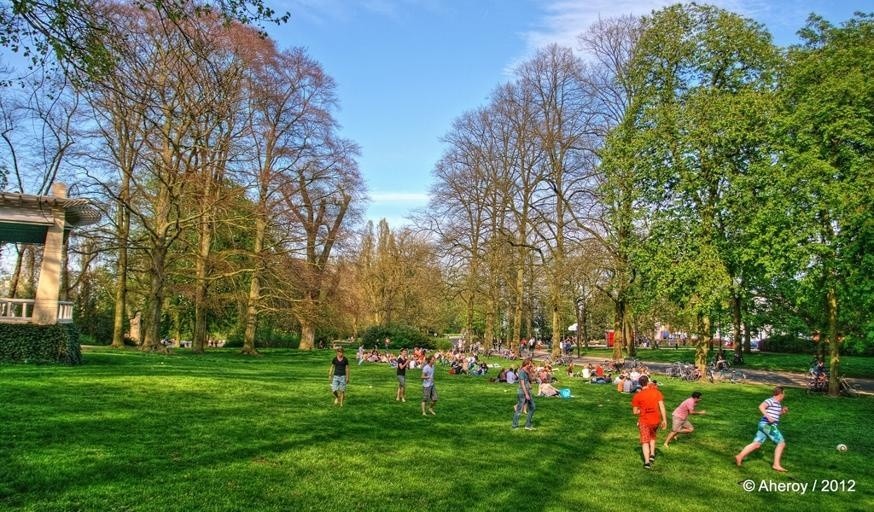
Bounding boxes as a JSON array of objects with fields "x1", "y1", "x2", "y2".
[
  {"x1": 835, "y1": 370, "x2": 861, "y2": 399},
  {"x1": 805, "y1": 373, "x2": 831, "y2": 394},
  {"x1": 487, "y1": 348, "x2": 750, "y2": 386}
]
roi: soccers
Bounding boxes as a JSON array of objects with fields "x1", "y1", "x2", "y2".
[{"x1": 836, "y1": 443, "x2": 846, "y2": 451}]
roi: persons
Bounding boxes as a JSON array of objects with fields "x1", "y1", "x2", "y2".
[
  {"x1": 663, "y1": 392, "x2": 706, "y2": 448},
  {"x1": 632, "y1": 376, "x2": 667, "y2": 468},
  {"x1": 327, "y1": 346, "x2": 349, "y2": 406},
  {"x1": 808, "y1": 359, "x2": 827, "y2": 391},
  {"x1": 395, "y1": 348, "x2": 410, "y2": 402},
  {"x1": 734, "y1": 387, "x2": 789, "y2": 471},
  {"x1": 511, "y1": 357, "x2": 537, "y2": 430},
  {"x1": 160, "y1": 335, "x2": 218, "y2": 348},
  {"x1": 318, "y1": 336, "x2": 726, "y2": 396},
  {"x1": 420, "y1": 355, "x2": 438, "y2": 416},
  {"x1": 512, "y1": 356, "x2": 534, "y2": 414}
]
